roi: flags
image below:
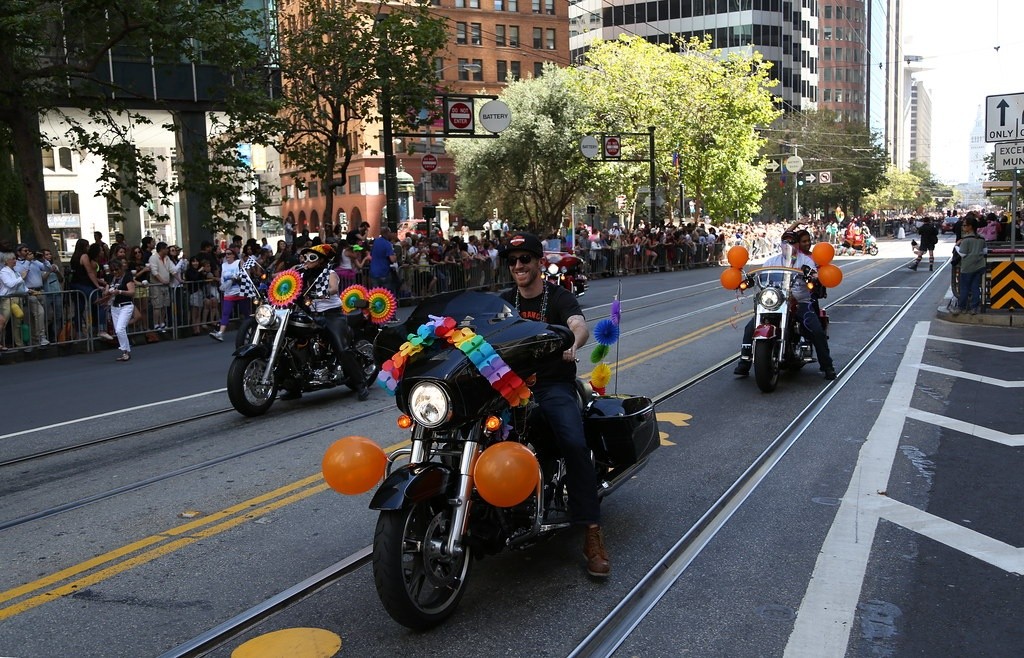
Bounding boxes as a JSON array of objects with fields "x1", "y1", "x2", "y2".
[
  {"x1": 240, "y1": 269, "x2": 256, "y2": 298},
  {"x1": 243, "y1": 257, "x2": 255, "y2": 269},
  {"x1": 313, "y1": 262, "x2": 331, "y2": 299}
]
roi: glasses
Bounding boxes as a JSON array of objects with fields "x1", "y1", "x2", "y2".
[
  {"x1": 109, "y1": 268, "x2": 119, "y2": 272},
  {"x1": 963, "y1": 224, "x2": 969, "y2": 227},
  {"x1": 19, "y1": 250, "x2": 30, "y2": 252},
  {"x1": 505, "y1": 254, "x2": 535, "y2": 267},
  {"x1": 224, "y1": 253, "x2": 233, "y2": 255},
  {"x1": 782, "y1": 239, "x2": 796, "y2": 246},
  {"x1": 299, "y1": 252, "x2": 322, "y2": 264}
]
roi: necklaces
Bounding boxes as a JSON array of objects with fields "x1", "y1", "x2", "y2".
[{"x1": 515, "y1": 280, "x2": 548, "y2": 322}]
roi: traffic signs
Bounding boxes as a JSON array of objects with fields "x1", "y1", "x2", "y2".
[{"x1": 985, "y1": 91, "x2": 1024, "y2": 143}]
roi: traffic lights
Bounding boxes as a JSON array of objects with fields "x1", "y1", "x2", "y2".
[{"x1": 797, "y1": 170, "x2": 806, "y2": 189}]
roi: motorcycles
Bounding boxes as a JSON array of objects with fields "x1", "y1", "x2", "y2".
[
  {"x1": 366, "y1": 289, "x2": 662, "y2": 633},
  {"x1": 538, "y1": 237, "x2": 590, "y2": 299},
  {"x1": 836, "y1": 229, "x2": 879, "y2": 257},
  {"x1": 225, "y1": 259, "x2": 404, "y2": 419},
  {"x1": 737, "y1": 240, "x2": 831, "y2": 394}
]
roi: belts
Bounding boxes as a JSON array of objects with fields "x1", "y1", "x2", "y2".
[{"x1": 113, "y1": 303, "x2": 131, "y2": 307}]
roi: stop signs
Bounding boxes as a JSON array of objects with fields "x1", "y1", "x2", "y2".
[
  {"x1": 605, "y1": 137, "x2": 620, "y2": 157},
  {"x1": 422, "y1": 154, "x2": 438, "y2": 171},
  {"x1": 447, "y1": 101, "x2": 473, "y2": 130}
]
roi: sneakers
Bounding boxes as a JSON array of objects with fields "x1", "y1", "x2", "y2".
[
  {"x1": 40, "y1": 336, "x2": 50, "y2": 346},
  {"x1": 209, "y1": 330, "x2": 224, "y2": 343}
]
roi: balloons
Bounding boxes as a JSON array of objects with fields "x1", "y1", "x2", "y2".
[
  {"x1": 474, "y1": 440, "x2": 540, "y2": 508},
  {"x1": 813, "y1": 242, "x2": 834, "y2": 265},
  {"x1": 721, "y1": 268, "x2": 742, "y2": 289},
  {"x1": 728, "y1": 246, "x2": 748, "y2": 269},
  {"x1": 322, "y1": 437, "x2": 388, "y2": 495},
  {"x1": 818, "y1": 264, "x2": 842, "y2": 287}
]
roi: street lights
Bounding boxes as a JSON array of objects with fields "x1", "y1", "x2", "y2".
[{"x1": 421, "y1": 62, "x2": 482, "y2": 238}]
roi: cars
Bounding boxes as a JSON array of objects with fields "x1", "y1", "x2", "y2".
[{"x1": 941, "y1": 216, "x2": 959, "y2": 234}]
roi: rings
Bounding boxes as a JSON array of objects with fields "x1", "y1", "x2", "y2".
[{"x1": 568, "y1": 349, "x2": 571, "y2": 350}]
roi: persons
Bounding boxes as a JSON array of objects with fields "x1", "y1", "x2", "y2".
[
  {"x1": 0, "y1": 210, "x2": 1024, "y2": 361},
  {"x1": 280, "y1": 244, "x2": 370, "y2": 399},
  {"x1": 908, "y1": 217, "x2": 938, "y2": 272},
  {"x1": 955, "y1": 217, "x2": 987, "y2": 314},
  {"x1": 733, "y1": 231, "x2": 836, "y2": 380},
  {"x1": 441, "y1": 233, "x2": 611, "y2": 577}
]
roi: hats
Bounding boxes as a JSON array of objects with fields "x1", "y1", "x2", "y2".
[
  {"x1": 497, "y1": 231, "x2": 544, "y2": 259},
  {"x1": 780, "y1": 230, "x2": 800, "y2": 243},
  {"x1": 612, "y1": 222, "x2": 618, "y2": 227}
]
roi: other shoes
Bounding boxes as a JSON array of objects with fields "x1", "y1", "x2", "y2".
[
  {"x1": 962, "y1": 308, "x2": 977, "y2": 316},
  {"x1": 116, "y1": 353, "x2": 130, "y2": 361},
  {"x1": 952, "y1": 307, "x2": 967, "y2": 317},
  {"x1": 357, "y1": 379, "x2": 369, "y2": 401},
  {"x1": 16, "y1": 345, "x2": 31, "y2": 354},
  {"x1": 153, "y1": 323, "x2": 168, "y2": 333},
  {"x1": 824, "y1": 367, "x2": 837, "y2": 381},
  {"x1": 581, "y1": 527, "x2": 612, "y2": 578},
  {"x1": 733, "y1": 359, "x2": 752, "y2": 376},
  {"x1": 908, "y1": 264, "x2": 918, "y2": 271},
  {"x1": 0, "y1": 344, "x2": 9, "y2": 351},
  {"x1": 96, "y1": 332, "x2": 114, "y2": 341},
  {"x1": 929, "y1": 265, "x2": 933, "y2": 271},
  {"x1": 279, "y1": 391, "x2": 303, "y2": 401}
]
